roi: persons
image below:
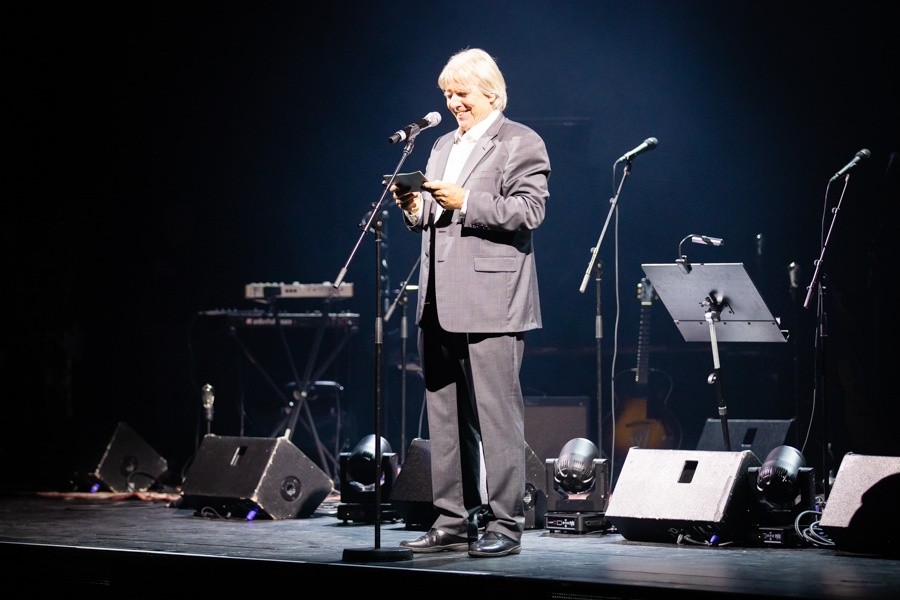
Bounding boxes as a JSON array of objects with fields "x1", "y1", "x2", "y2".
[{"x1": 389, "y1": 47, "x2": 550, "y2": 556}]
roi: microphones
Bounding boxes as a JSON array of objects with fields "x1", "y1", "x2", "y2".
[
  {"x1": 618, "y1": 137, "x2": 658, "y2": 164},
  {"x1": 831, "y1": 148, "x2": 872, "y2": 183},
  {"x1": 389, "y1": 111, "x2": 441, "y2": 144}
]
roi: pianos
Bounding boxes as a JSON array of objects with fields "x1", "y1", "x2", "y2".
[{"x1": 195, "y1": 306, "x2": 362, "y2": 502}]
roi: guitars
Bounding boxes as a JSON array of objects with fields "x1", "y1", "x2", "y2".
[{"x1": 597, "y1": 276, "x2": 684, "y2": 466}]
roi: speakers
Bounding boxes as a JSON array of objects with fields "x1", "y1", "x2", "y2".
[
  {"x1": 818, "y1": 451, "x2": 900, "y2": 561},
  {"x1": 389, "y1": 437, "x2": 548, "y2": 530},
  {"x1": 604, "y1": 445, "x2": 763, "y2": 547},
  {"x1": 696, "y1": 417, "x2": 813, "y2": 464},
  {"x1": 181, "y1": 434, "x2": 335, "y2": 520},
  {"x1": 520, "y1": 395, "x2": 591, "y2": 466},
  {"x1": 75, "y1": 422, "x2": 168, "y2": 495}
]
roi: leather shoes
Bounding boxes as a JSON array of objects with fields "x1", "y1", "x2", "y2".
[
  {"x1": 399, "y1": 528, "x2": 479, "y2": 551},
  {"x1": 468, "y1": 531, "x2": 521, "y2": 555}
]
